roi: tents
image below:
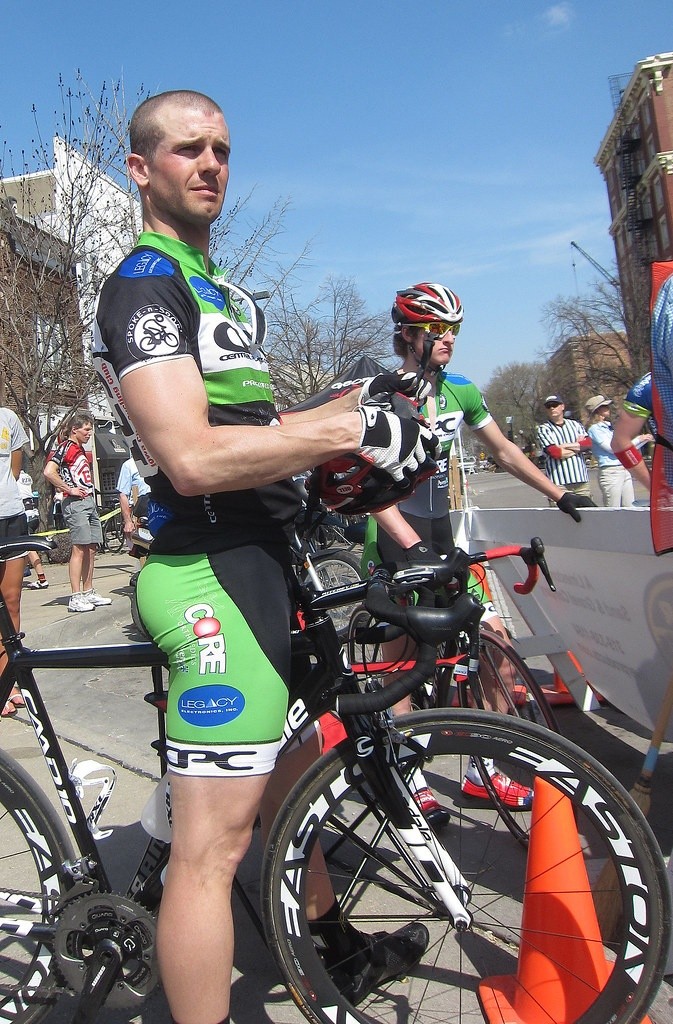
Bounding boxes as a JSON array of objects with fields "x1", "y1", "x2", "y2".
[{"x1": 279, "y1": 356, "x2": 468, "y2": 509}]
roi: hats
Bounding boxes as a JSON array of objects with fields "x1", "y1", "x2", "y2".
[
  {"x1": 584, "y1": 395, "x2": 614, "y2": 418},
  {"x1": 543, "y1": 394, "x2": 563, "y2": 403}
]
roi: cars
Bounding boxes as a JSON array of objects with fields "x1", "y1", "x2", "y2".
[{"x1": 457, "y1": 457, "x2": 476, "y2": 473}]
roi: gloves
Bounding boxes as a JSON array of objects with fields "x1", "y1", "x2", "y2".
[
  {"x1": 352, "y1": 404, "x2": 442, "y2": 489},
  {"x1": 404, "y1": 541, "x2": 452, "y2": 586},
  {"x1": 358, "y1": 372, "x2": 432, "y2": 413},
  {"x1": 556, "y1": 493, "x2": 597, "y2": 522}
]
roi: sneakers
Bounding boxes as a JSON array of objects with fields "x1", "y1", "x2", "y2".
[
  {"x1": 81, "y1": 588, "x2": 112, "y2": 606},
  {"x1": 460, "y1": 773, "x2": 533, "y2": 812},
  {"x1": 413, "y1": 786, "x2": 450, "y2": 833},
  {"x1": 67, "y1": 592, "x2": 95, "y2": 612},
  {"x1": 27, "y1": 579, "x2": 49, "y2": 589},
  {"x1": 335, "y1": 922, "x2": 429, "y2": 1007}
]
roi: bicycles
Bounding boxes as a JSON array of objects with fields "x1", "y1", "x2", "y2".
[{"x1": 0, "y1": 498, "x2": 673, "y2": 1024}]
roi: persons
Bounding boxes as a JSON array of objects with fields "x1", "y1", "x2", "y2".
[
  {"x1": 361, "y1": 281, "x2": 598, "y2": 830},
  {"x1": 536, "y1": 394, "x2": 656, "y2": 508},
  {"x1": 610, "y1": 372, "x2": 657, "y2": 493},
  {"x1": 90, "y1": 91, "x2": 442, "y2": 1024},
  {"x1": 44, "y1": 415, "x2": 111, "y2": 612},
  {"x1": 115, "y1": 455, "x2": 151, "y2": 568},
  {"x1": 0, "y1": 406, "x2": 49, "y2": 719}
]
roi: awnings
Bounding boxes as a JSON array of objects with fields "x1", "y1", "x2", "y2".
[{"x1": 93, "y1": 433, "x2": 130, "y2": 460}]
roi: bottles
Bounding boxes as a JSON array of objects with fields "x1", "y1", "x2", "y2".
[{"x1": 139, "y1": 775, "x2": 168, "y2": 842}]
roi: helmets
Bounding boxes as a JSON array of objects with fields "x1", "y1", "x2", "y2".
[
  {"x1": 392, "y1": 282, "x2": 464, "y2": 324},
  {"x1": 303, "y1": 385, "x2": 440, "y2": 515}
]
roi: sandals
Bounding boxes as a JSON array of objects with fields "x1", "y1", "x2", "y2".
[{"x1": 1, "y1": 687, "x2": 25, "y2": 717}]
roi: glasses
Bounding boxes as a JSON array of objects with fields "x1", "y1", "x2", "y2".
[
  {"x1": 401, "y1": 321, "x2": 460, "y2": 338},
  {"x1": 545, "y1": 402, "x2": 561, "y2": 409}
]
